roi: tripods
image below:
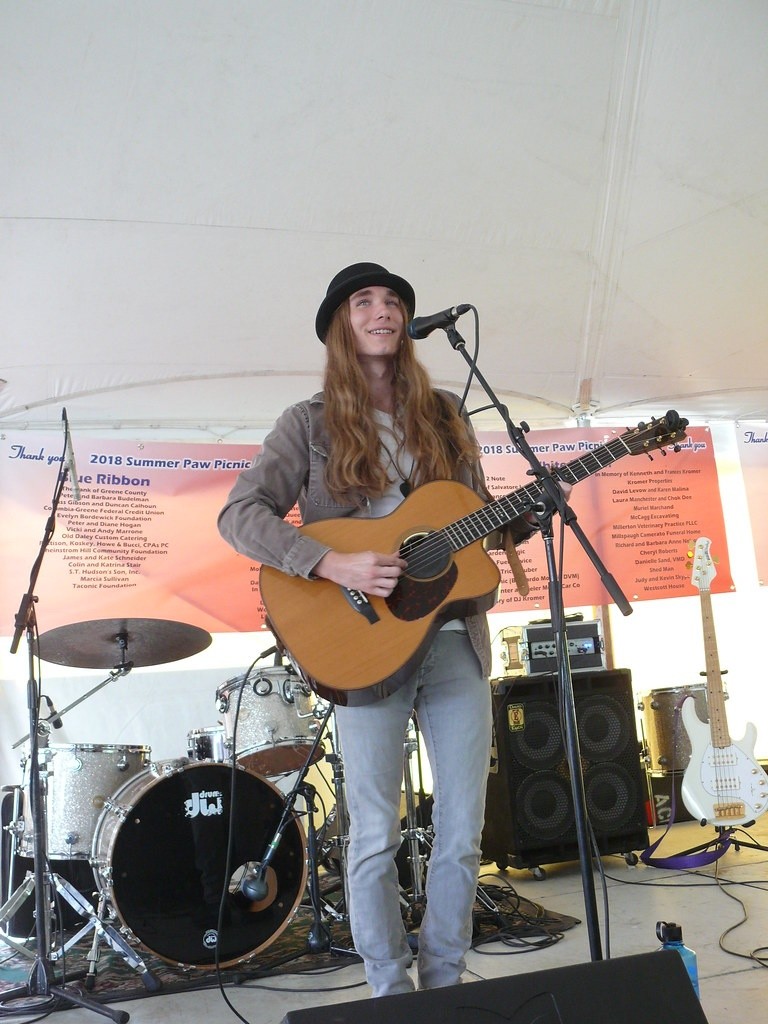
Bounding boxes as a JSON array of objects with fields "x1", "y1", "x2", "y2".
[
  {"x1": 0, "y1": 463, "x2": 165, "y2": 1024},
  {"x1": 302, "y1": 750, "x2": 350, "y2": 927},
  {"x1": 397, "y1": 723, "x2": 511, "y2": 932},
  {"x1": 233, "y1": 697, "x2": 361, "y2": 988}
]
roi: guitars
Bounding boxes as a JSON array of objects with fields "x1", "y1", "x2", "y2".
[
  {"x1": 256, "y1": 410, "x2": 690, "y2": 708},
  {"x1": 681, "y1": 537, "x2": 768, "y2": 826}
]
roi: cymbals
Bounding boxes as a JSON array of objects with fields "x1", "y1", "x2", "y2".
[{"x1": 31, "y1": 618, "x2": 212, "y2": 669}]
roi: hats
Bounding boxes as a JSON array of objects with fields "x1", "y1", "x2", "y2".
[{"x1": 315, "y1": 262, "x2": 415, "y2": 345}]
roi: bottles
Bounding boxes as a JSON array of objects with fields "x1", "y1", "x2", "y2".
[{"x1": 654, "y1": 921, "x2": 700, "y2": 1002}]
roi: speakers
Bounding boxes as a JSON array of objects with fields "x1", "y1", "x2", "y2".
[
  {"x1": 481, "y1": 669, "x2": 650, "y2": 869},
  {"x1": 280, "y1": 949, "x2": 709, "y2": 1024}
]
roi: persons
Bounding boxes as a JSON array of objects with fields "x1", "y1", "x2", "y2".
[{"x1": 216, "y1": 263, "x2": 572, "y2": 998}]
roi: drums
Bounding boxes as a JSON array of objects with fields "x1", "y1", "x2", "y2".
[
  {"x1": 214, "y1": 663, "x2": 326, "y2": 777},
  {"x1": 186, "y1": 727, "x2": 230, "y2": 761},
  {"x1": 91, "y1": 757, "x2": 310, "y2": 970},
  {"x1": 16, "y1": 744, "x2": 154, "y2": 861}
]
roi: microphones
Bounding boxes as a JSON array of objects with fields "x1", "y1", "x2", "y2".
[
  {"x1": 61, "y1": 412, "x2": 81, "y2": 500},
  {"x1": 242, "y1": 877, "x2": 269, "y2": 901},
  {"x1": 42, "y1": 696, "x2": 63, "y2": 730},
  {"x1": 274, "y1": 650, "x2": 282, "y2": 666},
  {"x1": 407, "y1": 305, "x2": 471, "y2": 340}
]
